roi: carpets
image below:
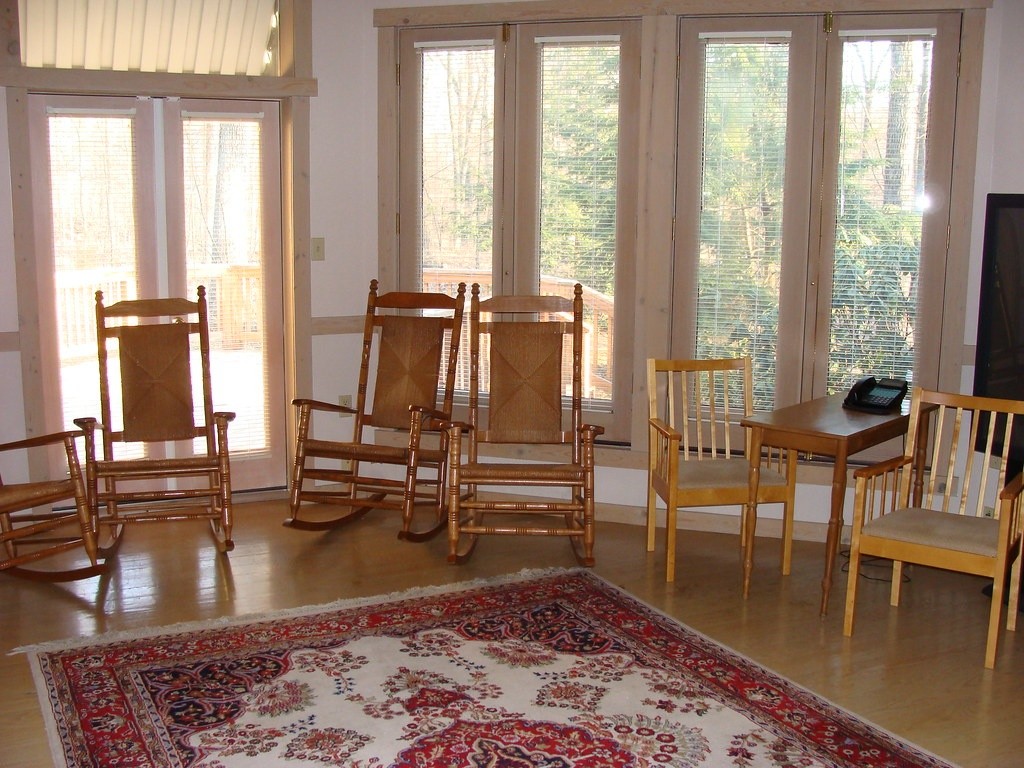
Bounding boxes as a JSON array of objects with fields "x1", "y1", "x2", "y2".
[{"x1": 10, "y1": 568, "x2": 959, "y2": 768}]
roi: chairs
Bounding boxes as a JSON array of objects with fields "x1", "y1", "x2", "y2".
[
  {"x1": 73, "y1": 286, "x2": 236, "y2": 560},
  {"x1": 646, "y1": 356, "x2": 797, "y2": 583},
  {"x1": 281, "y1": 279, "x2": 468, "y2": 545},
  {"x1": 843, "y1": 386, "x2": 1024, "y2": 668},
  {"x1": 0, "y1": 432, "x2": 107, "y2": 583},
  {"x1": 431, "y1": 283, "x2": 605, "y2": 569}
]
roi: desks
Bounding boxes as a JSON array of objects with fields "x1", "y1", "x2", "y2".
[{"x1": 740, "y1": 392, "x2": 939, "y2": 617}]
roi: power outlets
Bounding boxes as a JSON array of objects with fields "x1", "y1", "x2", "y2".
[
  {"x1": 983, "y1": 506, "x2": 994, "y2": 519},
  {"x1": 989, "y1": 455, "x2": 1002, "y2": 470},
  {"x1": 338, "y1": 395, "x2": 353, "y2": 418}
]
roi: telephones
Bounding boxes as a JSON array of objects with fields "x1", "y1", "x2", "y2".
[{"x1": 842, "y1": 375, "x2": 909, "y2": 414}]
